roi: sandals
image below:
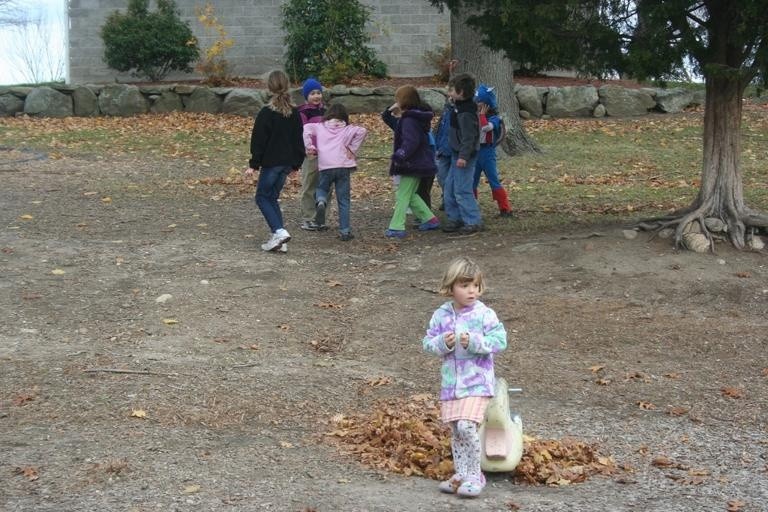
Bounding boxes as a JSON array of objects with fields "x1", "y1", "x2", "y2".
[
  {"x1": 457, "y1": 472, "x2": 486, "y2": 496},
  {"x1": 438, "y1": 473, "x2": 463, "y2": 494}
]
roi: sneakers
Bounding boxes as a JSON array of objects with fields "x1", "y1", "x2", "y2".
[
  {"x1": 316, "y1": 202, "x2": 325, "y2": 224},
  {"x1": 385, "y1": 229, "x2": 408, "y2": 238},
  {"x1": 340, "y1": 231, "x2": 354, "y2": 240},
  {"x1": 415, "y1": 216, "x2": 485, "y2": 235},
  {"x1": 500, "y1": 209, "x2": 513, "y2": 217},
  {"x1": 261, "y1": 229, "x2": 291, "y2": 253},
  {"x1": 301, "y1": 220, "x2": 328, "y2": 231}
]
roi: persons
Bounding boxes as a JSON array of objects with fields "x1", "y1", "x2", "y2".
[
  {"x1": 472, "y1": 84, "x2": 513, "y2": 218},
  {"x1": 384, "y1": 86, "x2": 440, "y2": 239},
  {"x1": 443, "y1": 75, "x2": 484, "y2": 234},
  {"x1": 410, "y1": 102, "x2": 436, "y2": 226},
  {"x1": 295, "y1": 78, "x2": 329, "y2": 231},
  {"x1": 246, "y1": 70, "x2": 304, "y2": 252},
  {"x1": 303, "y1": 104, "x2": 366, "y2": 241},
  {"x1": 423, "y1": 256, "x2": 506, "y2": 497},
  {"x1": 435, "y1": 81, "x2": 455, "y2": 210}
]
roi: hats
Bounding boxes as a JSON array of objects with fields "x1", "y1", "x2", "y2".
[{"x1": 304, "y1": 78, "x2": 322, "y2": 100}]
